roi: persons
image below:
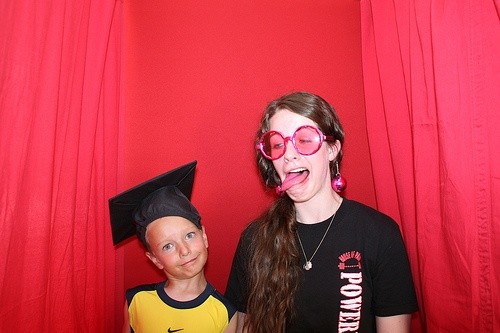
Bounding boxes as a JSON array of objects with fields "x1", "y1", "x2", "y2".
[
  {"x1": 222, "y1": 90, "x2": 420, "y2": 333},
  {"x1": 123, "y1": 183, "x2": 237, "y2": 332}
]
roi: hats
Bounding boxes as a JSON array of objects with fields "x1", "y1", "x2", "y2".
[{"x1": 108, "y1": 160, "x2": 200, "y2": 246}]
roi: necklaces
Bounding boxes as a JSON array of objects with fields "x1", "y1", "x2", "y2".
[{"x1": 295, "y1": 195, "x2": 342, "y2": 270}]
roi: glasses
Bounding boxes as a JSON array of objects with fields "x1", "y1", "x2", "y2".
[{"x1": 255, "y1": 125, "x2": 335, "y2": 160}]
